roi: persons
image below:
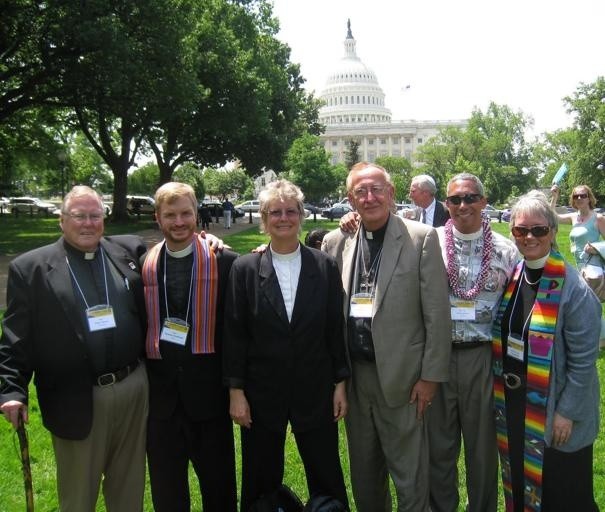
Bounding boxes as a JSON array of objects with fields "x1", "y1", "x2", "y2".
[
  {"x1": 252, "y1": 162, "x2": 451, "y2": 511},
  {"x1": 549, "y1": 184, "x2": 605, "y2": 353},
  {"x1": 493, "y1": 190, "x2": 601, "y2": 511},
  {"x1": 400, "y1": 176, "x2": 451, "y2": 228},
  {"x1": 126, "y1": 192, "x2": 342, "y2": 230},
  {"x1": 339, "y1": 172, "x2": 520, "y2": 512},
  {"x1": 138, "y1": 180, "x2": 239, "y2": 512},
  {"x1": 226, "y1": 179, "x2": 350, "y2": 511},
  {"x1": 0, "y1": 186, "x2": 233, "y2": 512},
  {"x1": 305, "y1": 226, "x2": 331, "y2": 253}
]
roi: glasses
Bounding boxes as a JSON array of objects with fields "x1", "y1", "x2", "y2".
[
  {"x1": 572, "y1": 193, "x2": 590, "y2": 199},
  {"x1": 353, "y1": 184, "x2": 387, "y2": 195},
  {"x1": 63, "y1": 211, "x2": 106, "y2": 221},
  {"x1": 512, "y1": 225, "x2": 551, "y2": 237},
  {"x1": 264, "y1": 207, "x2": 300, "y2": 217},
  {"x1": 445, "y1": 193, "x2": 483, "y2": 205}
]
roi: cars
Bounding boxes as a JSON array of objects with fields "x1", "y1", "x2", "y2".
[
  {"x1": 302, "y1": 197, "x2": 352, "y2": 218},
  {"x1": 481, "y1": 204, "x2": 511, "y2": 221},
  {"x1": 235, "y1": 200, "x2": 261, "y2": 212},
  {"x1": 562, "y1": 206, "x2": 604, "y2": 216},
  {"x1": 395, "y1": 204, "x2": 415, "y2": 212},
  {"x1": 203, "y1": 204, "x2": 245, "y2": 217},
  {"x1": 0, "y1": 197, "x2": 10, "y2": 209}
]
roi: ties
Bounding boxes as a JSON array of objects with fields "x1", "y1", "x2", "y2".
[{"x1": 421, "y1": 209, "x2": 427, "y2": 223}]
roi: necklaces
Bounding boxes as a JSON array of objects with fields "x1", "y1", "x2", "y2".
[
  {"x1": 523, "y1": 264, "x2": 544, "y2": 285},
  {"x1": 358, "y1": 223, "x2": 383, "y2": 299},
  {"x1": 574, "y1": 210, "x2": 589, "y2": 223}
]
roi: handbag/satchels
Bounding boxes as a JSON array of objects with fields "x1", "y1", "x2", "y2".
[{"x1": 579, "y1": 264, "x2": 605, "y2": 301}]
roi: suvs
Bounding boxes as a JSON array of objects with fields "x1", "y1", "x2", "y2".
[
  {"x1": 8, "y1": 198, "x2": 56, "y2": 214},
  {"x1": 127, "y1": 196, "x2": 155, "y2": 214}
]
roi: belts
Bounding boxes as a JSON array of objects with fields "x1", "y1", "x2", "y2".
[
  {"x1": 452, "y1": 342, "x2": 476, "y2": 352},
  {"x1": 95, "y1": 357, "x2": 144, "y2": 388},
  {"x1": 502, "y1": 371, "x2": 528, "y2": 389}
]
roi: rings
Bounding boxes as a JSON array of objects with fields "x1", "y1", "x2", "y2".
[{"x1": 427, "y1": 399, "x2": 433, "y2": 407}]
